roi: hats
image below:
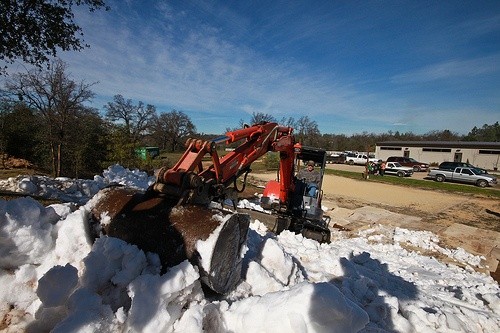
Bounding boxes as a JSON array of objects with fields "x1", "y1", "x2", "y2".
[{"x1": 308, "y1": 160, "x2": 315, "y2": 167}]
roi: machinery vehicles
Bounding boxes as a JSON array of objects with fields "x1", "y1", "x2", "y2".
[{"x1": 87, "y1": 121, "x2": 332, "y2": 296}]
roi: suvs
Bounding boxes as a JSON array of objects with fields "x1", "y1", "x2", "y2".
[
  {"x1": 384, "y1": 156, "x2": 429, "y2": 172},
  {"x1": 439, "y1": 161, "x2": 488, "y2": 175}
]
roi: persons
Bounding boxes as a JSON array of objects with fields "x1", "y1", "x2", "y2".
[
  {"x1": 296, "y1": 160, "x2": 320, "y2": 197},
  {"x1": 368, "y1": 160, "x2": 386, "y2": 176}
]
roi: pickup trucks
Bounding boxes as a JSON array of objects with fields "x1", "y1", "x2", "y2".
[
  {"x1": 325, "y1": 151, "x2": 356, "y2": 164},
  {"x1": 428, "y1": 166, "x2": 498, "y2": 188},
  {"x1": 346, "y1": 153, "x2": 382, "y2": 166},
  {"x1": 384, "y1": 162, "x2": 414, "y2": 177}
]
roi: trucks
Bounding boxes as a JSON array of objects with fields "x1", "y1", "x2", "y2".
[{"x1": 135, "y1": 146, "x2": 165, "y2": 164}]
